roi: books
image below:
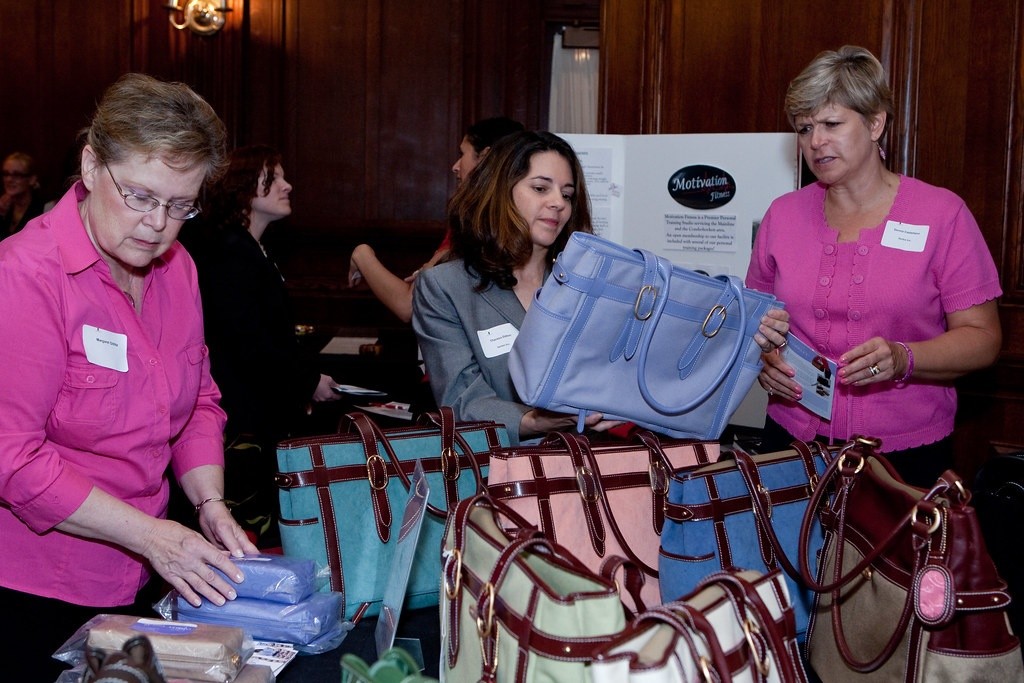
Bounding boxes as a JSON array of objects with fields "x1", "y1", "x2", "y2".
[{"x1": 780, "y1": 331, "x2": 837, "y2": 421}]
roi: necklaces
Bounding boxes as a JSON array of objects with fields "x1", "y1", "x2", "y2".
[{"x1": 123, "y1": 276, "x2": 135, "y2": 308}]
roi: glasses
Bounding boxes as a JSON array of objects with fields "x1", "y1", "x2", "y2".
[{"x1": 97, "y1": 149, "x2": 202, "y2": 221}]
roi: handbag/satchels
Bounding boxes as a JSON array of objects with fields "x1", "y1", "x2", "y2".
[
  {"x1": 657, "y1": 441, "x2": 837, "y2": 643},
  {"x1": 797, "y1": 434, "x2": 1024, "y2": 683},
  {"x1": 488, "y1": 431, "x2": 719, "y2": 619},
  {"x1": 274, "y1": 407, "x2": 510, "y2": 623},
  {"x1": 438, "y1": 495, "x2": 648, "y2": 683},
  {"x1": 591, "y1": 565, "x2": 809, "y2": 683},
  {"x1": 506, "y1": 232, "x2": 785, "y2": 440}
]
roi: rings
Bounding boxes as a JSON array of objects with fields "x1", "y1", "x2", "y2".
[
  {"x1": 766, "y1": 388, "x2": 773, "y2": 396},
  {"x1": 779, "y1": 341, "x2": 787, "y2": 347},
  {"x1": 865, "y1": 363, "x2": 880, "y2": 377}
]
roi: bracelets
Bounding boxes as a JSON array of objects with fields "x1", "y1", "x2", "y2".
[
  {"x1": 889, "y1": 342, "x2": 914, "y2": 383},
  {"x1": 195, "y1": 498, "x2": 228, "y2": 516}
]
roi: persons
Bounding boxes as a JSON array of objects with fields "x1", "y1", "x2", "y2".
[
  {"x1": 196, "y1": 147, "x2": 342, "y2": 472},
  {"x1": 0, "y1": 152, "x2": 47, "y2": 242},
  {"x1": 0, "y1": 74, "x2": 260, "y2": 683},
  {"x1": 346, "y1": 117, "x2": 526, "y2": 418},
  {"x1": 412, "y1": 131, "x2": 630, "y2": 443},
  {"x1": 746, "y1": 46, "x2": 1003, "y2": 490}
]
